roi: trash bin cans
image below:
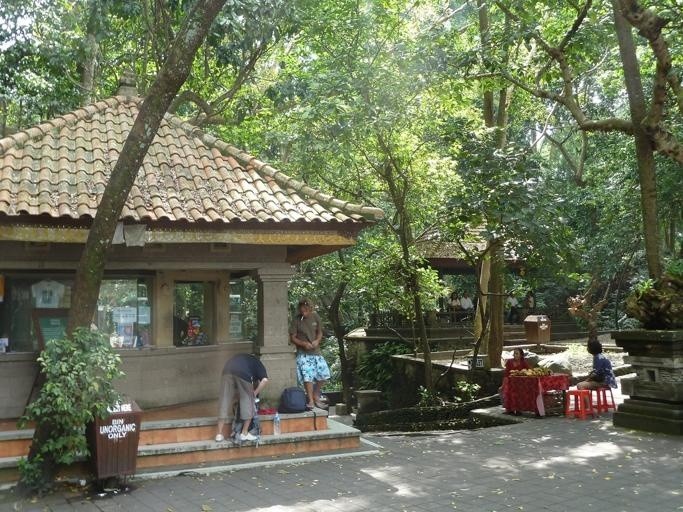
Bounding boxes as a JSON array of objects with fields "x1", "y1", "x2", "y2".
[
  {"x1": 466, "y1": 355, "x2": 489, "y2": 370},
  {"x1": 88, "y1": 398, "x2": 142, "y2": 492},
  {"x1": 523, "y1": 314, "x2": 550, "y2": 344}
]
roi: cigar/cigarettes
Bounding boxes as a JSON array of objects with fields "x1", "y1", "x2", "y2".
[{"x1": 300, "y1": 315, "x2": 303, "y2": 320}]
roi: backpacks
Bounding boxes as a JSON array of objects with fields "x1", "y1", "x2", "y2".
[
  {"x1": 277, "y1": 386, "x2": 308, "y2": 414},
  {"x1": 229, "y1": 400, "x2": 262, "y2": 437}
]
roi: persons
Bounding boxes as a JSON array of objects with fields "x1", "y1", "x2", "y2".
[
  {"x1": 172, "y1": 314, "x2": 189, "y2": 347},
  {"x1": 289, "y1": 295, "x2": 332, "y2": 410},
  {"x1": 576, "y1": 339, "x2": 618, "y2": 411},
  {"x1": 497, "y1": 347, "x2": 532, "y2": 417},
  {"x1": 215, "y1": 352, "x2": 269, "y2": 442},
  {"x1": 448, "y1": 289, "x2": 535, "y2": 325}
]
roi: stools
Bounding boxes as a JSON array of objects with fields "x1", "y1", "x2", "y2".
[{"x1": 564, "y1": 386, "x2": 615, "y2": 420}]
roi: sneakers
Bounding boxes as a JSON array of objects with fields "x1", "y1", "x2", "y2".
[
  {"x1": 240, "y1": 432, "x2": 258, "y2": 441},
  {"x1": 215, "y1": 433, "x2": 225, "y2": 442}
]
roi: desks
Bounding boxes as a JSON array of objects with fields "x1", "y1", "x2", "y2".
[{"x1": 508, "y1": 373, "x2": 569, "y2": 417}]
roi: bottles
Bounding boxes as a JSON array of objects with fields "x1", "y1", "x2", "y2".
[{"x1": 272, "y1": 412, "x2": 280, "y2": 437}]
